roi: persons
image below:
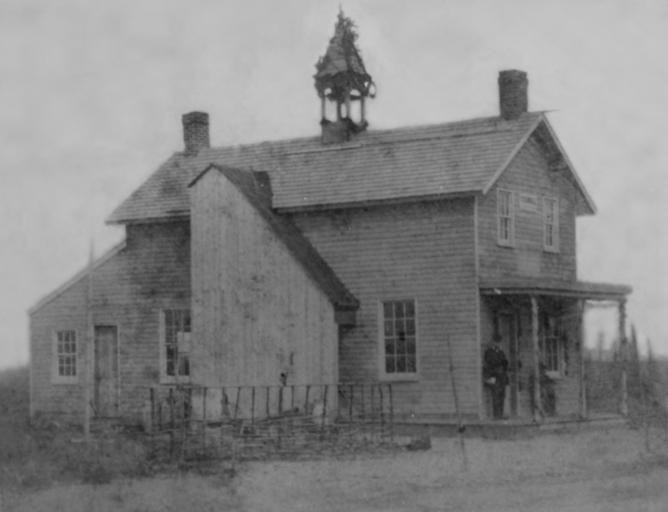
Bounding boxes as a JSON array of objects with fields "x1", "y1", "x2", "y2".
[{"x1": 484, "y1": 334, "x2": 509, "y2": 419}]
